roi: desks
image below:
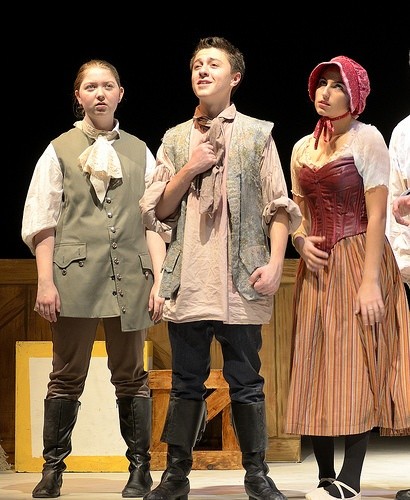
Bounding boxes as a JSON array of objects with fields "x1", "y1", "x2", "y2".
[{"x1": 0, "y1": 258, "x2": 305, "y2": 463}]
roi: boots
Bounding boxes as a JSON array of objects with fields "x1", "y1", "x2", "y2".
[
  {"x1": 32, "y1": 399, "x2": 80, "y2": 498},
  {"x1": 143, "y1": 399, "x2": 206, "y2": 500},
  {"x1": 116, "y1": 396, "x2": 153, "y2": 497},
  {"x1": 230, "y1": 400, "x2": 287, "y2": 500}
]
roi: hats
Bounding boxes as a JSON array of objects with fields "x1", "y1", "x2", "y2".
[{"x1": 309, "y1": 56, "x2": 370, "y2": 150}]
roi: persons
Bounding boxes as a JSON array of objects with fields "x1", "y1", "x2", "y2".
[
  {"x1": 21, "y1": 60, "x2": 166, "y2": 500},
  {"x1": 138, "y1": 36, "x2": 303, "y2": 500},
  {"x1": 385, "y1": 116, "x2": 410, "y2": 500},
  {"x1": 288, "y1": 55, "x2": 410, "y2": 500}
]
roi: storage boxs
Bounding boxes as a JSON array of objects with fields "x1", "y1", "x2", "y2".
[{"x1": 14, "y1": 340, "x2": 154, "y2": 474}]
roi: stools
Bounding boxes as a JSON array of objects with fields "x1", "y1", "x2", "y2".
[{"x1": 147, "y1": 368, "x2": 243, "y2": 471}]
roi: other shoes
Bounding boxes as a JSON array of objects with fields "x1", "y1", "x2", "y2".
[{"x1": 306, "y1": 478, "x2": 362, "y2": 500}]
